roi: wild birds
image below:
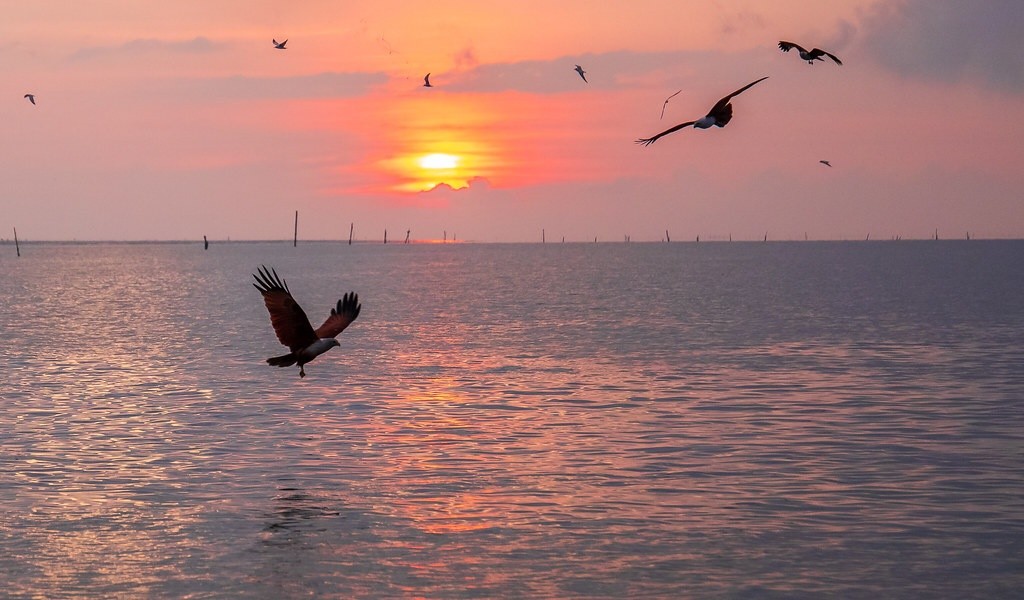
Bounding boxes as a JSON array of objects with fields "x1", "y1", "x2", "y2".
[
  {"x1": 272, "y1": 38, "x2": 288, "y2": 49},
  {"x1": 819, "y1": 160, "x2": 832, "y2": 167},
  {"x1": 24, "y1": 93, "x2": 36, "y2": 105},
  {"x1": 423, "y1": 73, "x2": 433, "y2": 87},
  {"x1": 660, "y1": 90, "x2": 682, "y2": 120},
  {"x1": 778, "y1": 40, "x2": 843, "y2": 67},
  {"x1": 573, "y1": 64, "x2": 588, "y2": 83},
  {"x1": 633, "y1": 76, "x2": 770, "y2": 148},
  {"x1": 252, "y1": 264, "x2": 361, "y2": 379}
]
